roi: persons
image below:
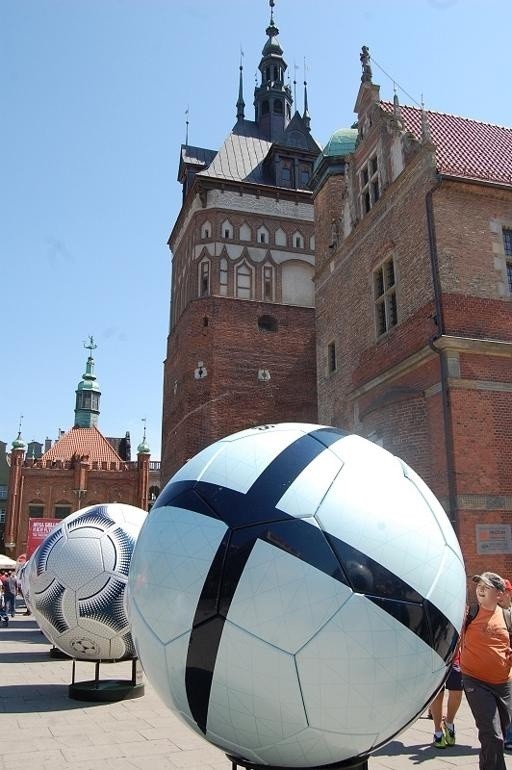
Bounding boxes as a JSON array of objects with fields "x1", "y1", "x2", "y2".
[
  {"x1": 452, "y1": 570, "x2": 512, "y2": 770},
  {"x1": 496, "y1": 578, "x2": 512, "y2": 612},
  {"x1": 0, "y1": 569, "x2": 31, "y2": 630},
  {"x1": 428, "y1": 643, "x2": 463, "y2": 748}
]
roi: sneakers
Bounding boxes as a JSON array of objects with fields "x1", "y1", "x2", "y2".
[{"x1": 433, "y1": 720, "x2": 456, "y2": 749}]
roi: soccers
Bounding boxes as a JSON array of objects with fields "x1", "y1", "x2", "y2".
[
  {"x1": 20, "y1": 503, "x2": 149, "y2": 665},
  {"x1": 124, "y1": 421, "x2": 467, "y2": 769}
]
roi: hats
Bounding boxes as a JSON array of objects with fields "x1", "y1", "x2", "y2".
[{"x1": 471, "y1": 570, "x2": 511, "y2": 595}]
